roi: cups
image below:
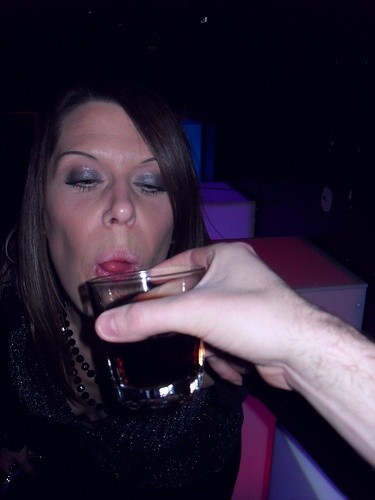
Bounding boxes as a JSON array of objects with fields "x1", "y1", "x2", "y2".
[{"x1": 78, "y1": 265, "x2": 206, "y2": 415}]
